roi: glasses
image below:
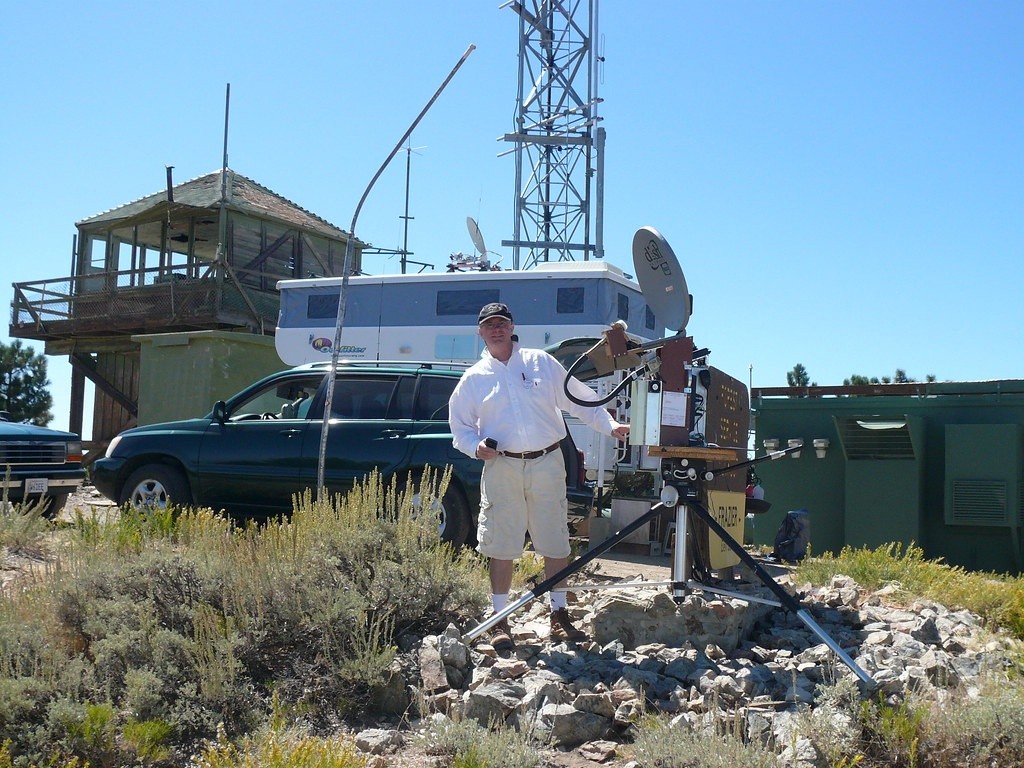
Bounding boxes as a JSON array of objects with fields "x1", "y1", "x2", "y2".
[{"x1": 480, "y1": 321, "x2": 512, "y2": 328}]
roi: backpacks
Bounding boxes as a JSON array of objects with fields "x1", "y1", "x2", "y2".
[{"x1": 771, "y1": 508, "x2": 811, "y2": 563}]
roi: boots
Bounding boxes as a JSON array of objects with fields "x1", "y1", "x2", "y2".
[
  {"x1": 550, "y1": 607, "x2": 587, "y2": 642},
  {"x1": 489, "y1": 611, "x2": 513, "y2": 649}
]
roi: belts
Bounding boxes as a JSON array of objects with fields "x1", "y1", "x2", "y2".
[{"x1": 499, "y1": 443, "x2": 560, "y2": 460}]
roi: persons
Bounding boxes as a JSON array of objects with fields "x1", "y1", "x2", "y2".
[{"x1": 449, "y1": 303, "x2": 631, "y2": 649}]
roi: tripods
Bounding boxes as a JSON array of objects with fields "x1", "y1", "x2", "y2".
[{"x1": 459, "y1": 463, "x2": 881, "y2": 694}]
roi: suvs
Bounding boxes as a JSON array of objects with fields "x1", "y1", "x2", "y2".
[{"x1": 91, "y1": 360, "x2": 594, "y2": 558}]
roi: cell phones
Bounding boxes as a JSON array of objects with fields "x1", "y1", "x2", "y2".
[{"x1": 486, "y1": 438, "x2": 498, "y2": 451}]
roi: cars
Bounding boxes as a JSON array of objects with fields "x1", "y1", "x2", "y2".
[{"x1": 0, "y1": 412, "x2": 87, "y2": 521}]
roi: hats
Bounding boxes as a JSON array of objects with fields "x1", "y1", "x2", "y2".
[{"x1": 477, "y1": 302, "x2": 512, "y2": 325}]
